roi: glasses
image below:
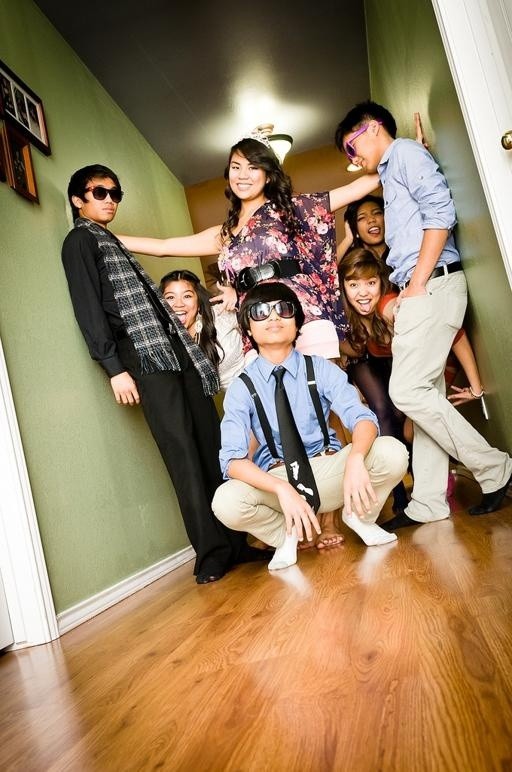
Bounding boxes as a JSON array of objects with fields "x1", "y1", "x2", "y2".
[
  {"x1": 345, "y1": 121, "x2": 384, "y2": 163},
  {"x1": 250, "y1": 299, "x2": 298, "y2": 320},
  {"x1": 84, "y1": 185, "x2": 125, "y2": 204}
]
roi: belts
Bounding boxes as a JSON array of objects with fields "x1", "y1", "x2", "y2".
[{"x1": 400, "y1": 260, "x2": 464, "y2": 290}]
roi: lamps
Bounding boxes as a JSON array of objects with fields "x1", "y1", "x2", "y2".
[{"x1": 251, "y1": 122, "x2": 293, "y2": 164}]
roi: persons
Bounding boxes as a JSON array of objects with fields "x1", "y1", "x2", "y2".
[{"x1": 61, "y1": 99, "x2": 512, "y2": 587}]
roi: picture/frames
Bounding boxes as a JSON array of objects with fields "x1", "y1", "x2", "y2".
[
  {"x1": 0, "y1": 60, "x2": 51, "y2": 157},
  {"x1": 0, "y1": 119, "x2": 39, "y2": 205}
]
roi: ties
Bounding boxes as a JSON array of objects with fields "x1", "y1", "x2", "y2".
[{"x1": 268, "y1": 368, "x2": 322, "y2": 518}]
